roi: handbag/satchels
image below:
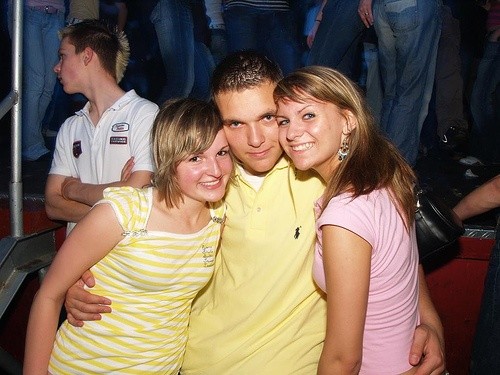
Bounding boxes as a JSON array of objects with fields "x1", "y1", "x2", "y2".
[{"x1": 412, "y1": 181, "x2": 466, "y2": 264}]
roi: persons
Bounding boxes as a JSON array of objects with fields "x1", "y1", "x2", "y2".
[
  {"x1": 95, "y1": 0, "x2": 216, "y2": 107},
  {"x1": 472, "y1": 1, "x2": 500, "y2": 153},
  {"x1": 1, "y1": 0, "x2": 67, "y2": 163},
  {"x1": 272, "y1": 66, "x2": 424, "y2": 375},
  {"x1": 434, "y1": 0, "x2": 488, "y2": 147},
  {"x1": 22, "y1": 97, "x2": 232, "y2": 375},
  {"x1": 64, "y1": 56, "x2": 447, "y2": 374},
  {"x1": 373, "y1": 0, "x2": 442, "y2": 162},
  {"x1": 46, "y1": 20, "x2": 164, "y2": 242},
  {"x1": 447, "y1": 168, "x2": 500, "y2": 375},
  {"x1": 305, "y1": 1, "x2": 373, "y2": 74},
  {"x1": 204, "y1": 0, "x2": 303, "y2": 74}
]
